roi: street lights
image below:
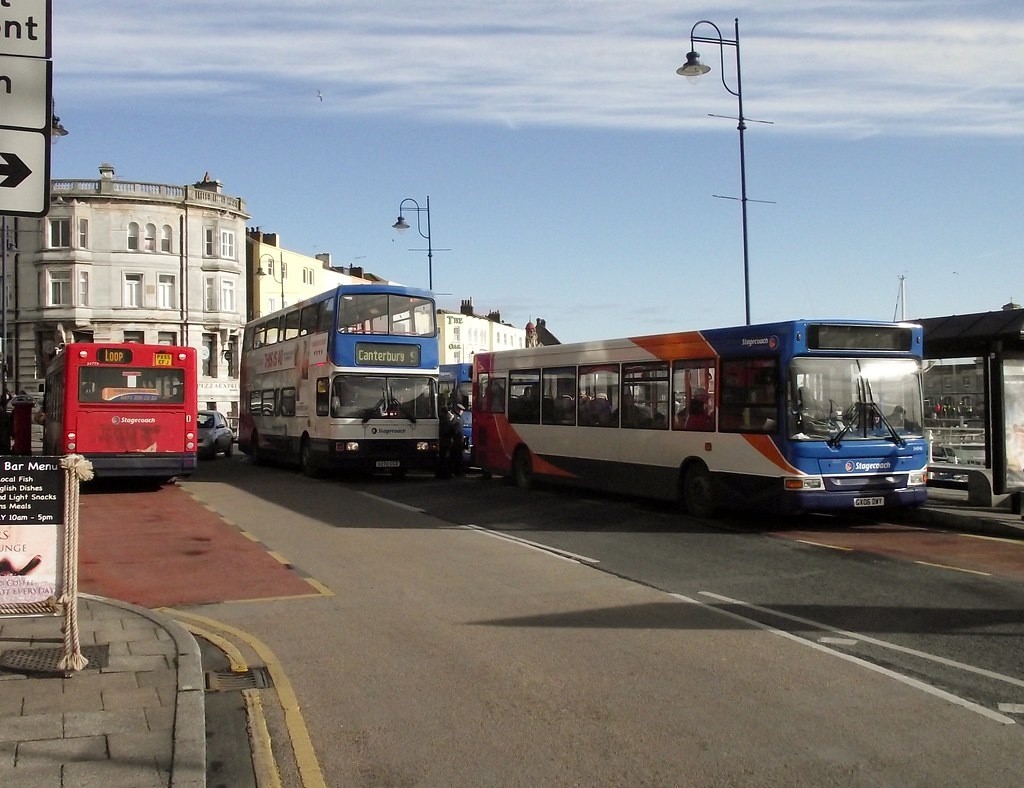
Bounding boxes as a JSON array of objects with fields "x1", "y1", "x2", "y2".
[
  {"x1": 676, "y1": 18, "x2": 777, "y2": 327},
  {"x1": 392, "y1": 195, "x2": 452, "y2": 290},
  {"x1": 255, "y1": 252, "x2": 284, "y2": 310}
]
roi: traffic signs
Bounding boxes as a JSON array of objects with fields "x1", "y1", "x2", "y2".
[{"x1": 0, "y1": 1, "x2": 54, "y2": 219}]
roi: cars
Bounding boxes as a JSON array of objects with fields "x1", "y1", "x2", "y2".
[
  {"x1": 931, "y1": 445, "x2": 961, "y2": 464},
  {"x1": 197, "y1": 409, "x2": 234, "y2": 461}
]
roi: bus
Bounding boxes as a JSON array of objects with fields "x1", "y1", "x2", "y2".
[
  {"x1": 474, "y1": 319, "x2": 929, "y2": 521},
  {"x1": 440, "y1": 362, "x2": 472, "y2": 469},
  {"x1": 238, "y1": 285, "x2": 440, "y2": 478},
  {"x1": 42, "y1": 342, "x2": 198, "y2": 494}
]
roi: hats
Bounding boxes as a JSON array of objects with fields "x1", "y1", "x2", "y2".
[
  {"x1": 694, "y1": 388, "x2": 711, "y2": 396},
  {"x1": 456, "y1": 404, "x2": 466, "y2": 410}
]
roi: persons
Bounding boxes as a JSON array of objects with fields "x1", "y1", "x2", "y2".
[
  {"x1": 931, "y1": 402, "x2": 975, "y2": 418},
  {"x1": 796, "y1": 386, "x2": 826, "y2": 421},
  {"x1": 579, "y1": 388, "x2": 711, "y2": 431},
  {"x1": 519, "y1": 386, "x2": 541, "y2": 410},
  {"x1": 884, "y1": 406, "x2": 906, "y2": 427},
  {"x1": 438, "y1": 404, "x2": 465, "y2": 479},
  {"x1": 206, "y1": 418, "x2": 214, "y2": 428}
]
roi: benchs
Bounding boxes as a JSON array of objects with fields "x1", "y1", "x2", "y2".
[{"x1": 927, "y1": 465, "x2": 1012, "y2": 508}]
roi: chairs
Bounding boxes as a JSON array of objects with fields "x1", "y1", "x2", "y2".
[{"x1": 510, "y1": 391, "x2": 659, "y2": 429}]
